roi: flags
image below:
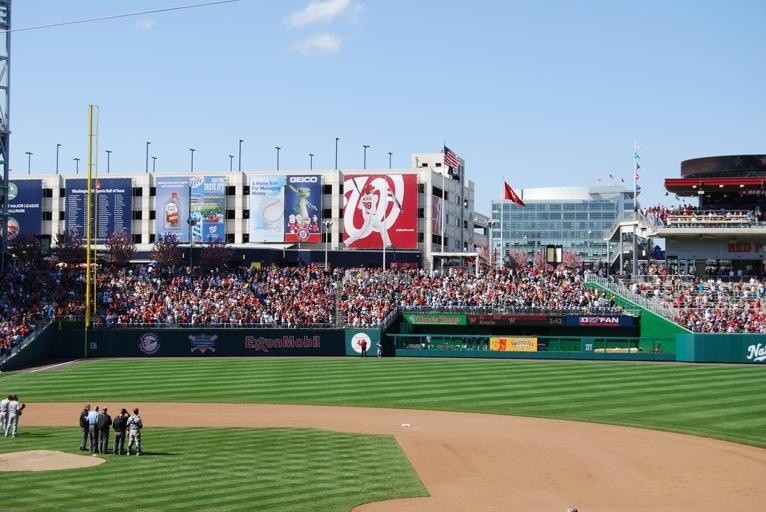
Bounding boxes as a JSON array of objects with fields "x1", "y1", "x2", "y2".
[
  {"x1": 503, "y1": 181, "x2": 526, "y2": 207},
  {"x1": 442, "y1": 144, "x2": 461, "y2": 169},
  {"x1": 634, "y1": 142, "x2": 642, "y2": 191}
]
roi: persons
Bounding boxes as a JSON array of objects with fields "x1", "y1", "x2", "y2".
[
  {"x1": 80, "y1": 403, "x2": 143, "y2": 456},
  {"x1": 638, "y1": 204, "x2": 766, "y2": 222},
  {"x1": 0, "y1": 394, "x2": 25, "y2": 438}
]
